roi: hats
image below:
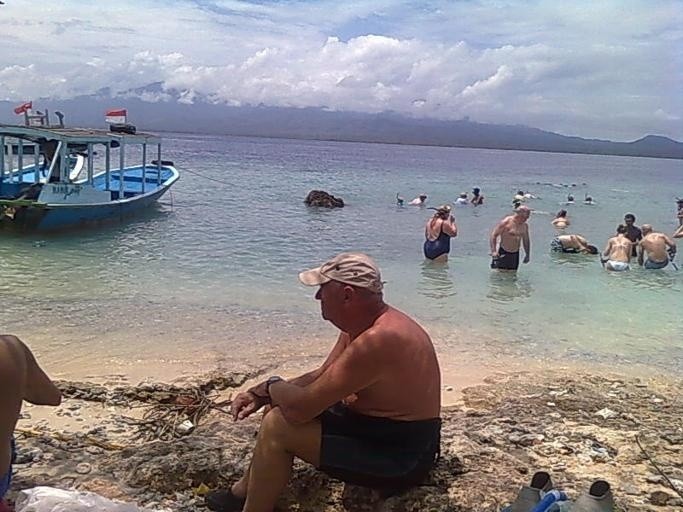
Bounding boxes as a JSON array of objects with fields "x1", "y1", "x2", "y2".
[
  {"x1": 298, "y1": 252, "x2": 383, "y2": 293},
  {"x1": 512, "y1": 205, "x2": 535, "y2": 212}
]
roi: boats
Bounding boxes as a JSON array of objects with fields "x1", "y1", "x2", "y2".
[{"x1": 0, "y1": 101, "x2": 180, "y2": 229}]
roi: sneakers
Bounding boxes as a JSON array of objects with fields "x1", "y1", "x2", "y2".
[
  {"x1": 509, "y1": 471, "x2": 551, "y2": 511},
  {"x1": 204, "y1": 488, "x2": 245, "y2": 512},
  {"x1": 566, "y1": 480, "x2": 614, "y2": 512}
]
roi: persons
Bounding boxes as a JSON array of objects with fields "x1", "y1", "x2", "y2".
[
  {"x1": 603, "y1": 224, "x2": 633, "y2": 272},
  {"x1": 424, "y1": 205, "x2": 457, "y2": 262},
  {"x1": 583, "y1": 196, "x2": 594, "y2": 204},
  {"x1": 491, "y1": 205, "x2": 530, "y2": 271},
  {"x1": 624, "y1": 214, "x2": 642, "y2": 257},
  {"x1": 205, "y1": 251, "x2": 441, "y2": 511},
  {"x1": 454, "y1": 192, "x2": 467, "y2": 205},
  {"x1": 0, "y1": 335, "x2": 62, "y2": 511},
  {"x1": 673, "y1": 224, "x2": 683, "y2": 238},
  {"x1": 525, "y1": 190, "x2": 541, "y2": 200},
  {"x1": 565, "y1": 193, "x2": 575, "y2": 205},
  {"x1": 551, "y1": 234, "x2": 597, "y2": 255},
  {"x1": 638, "y1": 224, "x2": 676, "y2": 269},
  {"x1": 513, "y1": 190, "x2": 524, "y2": 208},
  {"x1": 551, "y1": 209, "x2": 570, "y2": 229},
  {"x1": 675, "y1": 197, "x2": 683, "y2": 224},
  {"x1": 396, "y1": 192, "x2": 426, "y2": 205},
  {"x1": 470, "y1": 187, "x2": 484, "y2": 206}
]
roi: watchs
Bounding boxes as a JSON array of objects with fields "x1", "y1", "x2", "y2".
[{"x1": 266, "y1": 375, "x2": 283, "y2": 389}]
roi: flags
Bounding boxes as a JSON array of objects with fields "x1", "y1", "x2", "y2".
[
  {"x1": 105, "y1": 109, "x2": 127, "y2": 124},
  {"x1": 14, "y1": 101, "x2": 32, "y2": 114}
]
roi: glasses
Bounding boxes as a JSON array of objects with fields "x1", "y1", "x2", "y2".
[{"x1": 626, "y1": 220, "x2": 633, "y2": 223}]
